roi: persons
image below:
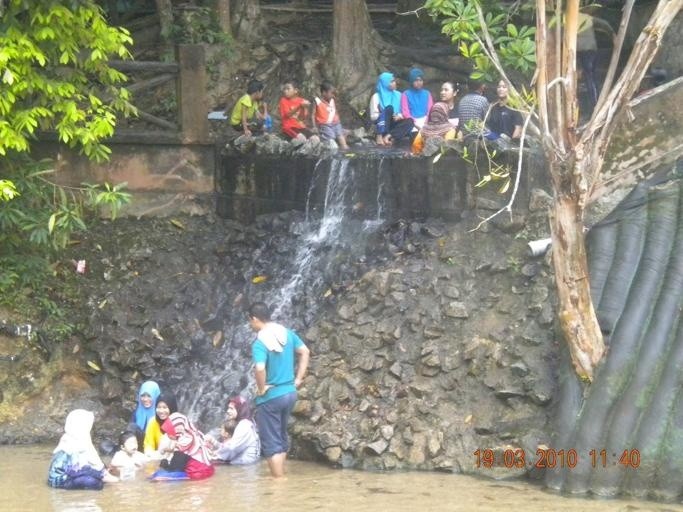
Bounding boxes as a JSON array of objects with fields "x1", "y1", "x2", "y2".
[
  {"x1": 246, "y1": 302, "x2": 310, "y2": 480},
  {"x1": 107, "y1": 380, "x2": 261, "y2": 481},
  {"x1": 229, "y1": 66, "x2": 522, "y2": 152},
  {"x1": 47, "y1": 409, "x2": 117, "y2": 492}
]
